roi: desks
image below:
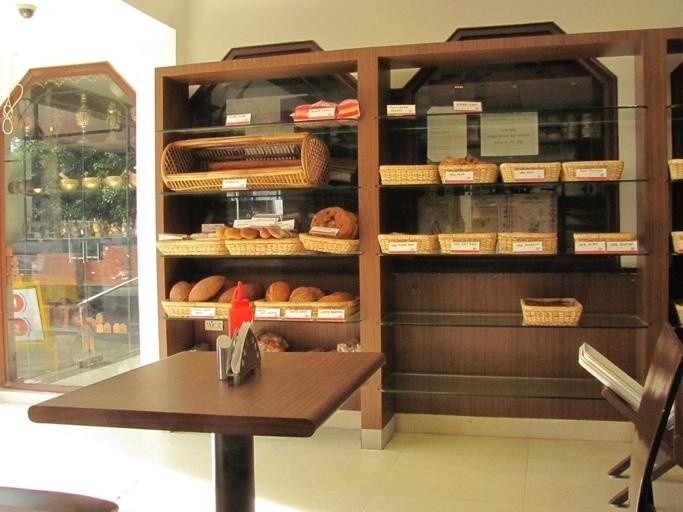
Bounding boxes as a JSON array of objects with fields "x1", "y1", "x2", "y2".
[{"x1": 28, "y1": 350, "x2": 386, "y2": 512}]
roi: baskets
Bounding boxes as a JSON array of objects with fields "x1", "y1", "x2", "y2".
[
  {"x1": 671, "y1": 231, "x2": 683, "y2": 254},
  {"x1": 161, "y1": 299, "x2": 360, "y2": 322},
  {"x1": 157, "y1": 232, "x2": 360, "y2": 256},
  {"x1": 667, "y1": 158, "x2": 683, "y2": 180},
  {"x1": 377, "y1": 230, "x2": 639, "y2": 255},
  {"x1": 520, "y1": 297, "x2": 584, "y2": 326},
  {"x1": 379, "y1": 160, "x2": 625, "y2": 186}
]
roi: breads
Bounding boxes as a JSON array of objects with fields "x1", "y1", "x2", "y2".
[
  {"x1": 86, "y1": 314, "x2": 129, "y2": 334},
  {"x1": 216, "y1": 225, "x2": 294, "y2": 239},
  {"x1": 169, "y1": 275, "x2": 355, "y2": 303},
  {"x1": 189, "y1": 332, "x2": 362, "y2": 352},
  {"x1": 310, "y1": 206, "x2": 358, "y2": 238}
]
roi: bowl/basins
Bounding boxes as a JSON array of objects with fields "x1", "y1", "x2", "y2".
[{"x1": 10, "y1": 174, "x2": 134, "y2": 194}]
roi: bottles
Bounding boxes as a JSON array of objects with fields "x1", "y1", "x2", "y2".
[
  {"x1": 579, "y1": 113, "x2": 593, "y2": 139},
  {"x1": 561, "y1": 114, "x2": 578, "y2": 138},
  {"x1": 227, "y1": 280, "x2": 252, "y2": 337},
  {"x1": 546, "y1": 117, "x2": 560, "y2": 138},
  {"x1": 216, "y1": 334, "x2": 232, "y2": 380}
]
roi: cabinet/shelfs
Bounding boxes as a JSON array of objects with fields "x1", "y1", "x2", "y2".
[
  {"x1": 23, "y1": 90, "x2": 130, "y2": 239},
  {"x1": 155, "y1": 25, "x2": 682, "y2": 449}
]
